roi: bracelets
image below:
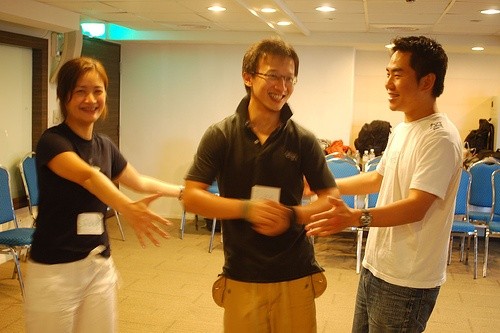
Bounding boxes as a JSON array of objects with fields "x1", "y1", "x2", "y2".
[
  {"x1": 288, "y1": 207, "x2": 296, "y2": 224},
  {"x1": 179, "y1": 189, "x2": 184, "y2": 200}
]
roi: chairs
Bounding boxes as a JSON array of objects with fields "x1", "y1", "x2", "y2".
[
  {"x1": 178, "y1": 180, "x2": 223, "y2": 252},
  {"x1": 306, "y1": 150, "x2": 500, "y2": 280},
  {"x1": 0, "y1": 165, "x2": 36, "y2": 298},
  {"x1": 16, "y1": 151, "x2": 39, "y2": 228}
]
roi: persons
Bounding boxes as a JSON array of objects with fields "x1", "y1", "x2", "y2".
[
  {"x1": 183, "y1": 39, "x2": 339, "y2": 333},
  {"x1": 304, "y1": 35, "x2": 463, "y2": 333},
  {"x1": 25, "y1": 58, "x2": 184, "y2": 333}
]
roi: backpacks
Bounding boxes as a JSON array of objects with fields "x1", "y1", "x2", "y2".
[
  {"x1": 464, "y1": 118, "x2": 495, "y2": 151},
  {"x1": 353, "y1": 120, "x2": 390, "y2": 158}
]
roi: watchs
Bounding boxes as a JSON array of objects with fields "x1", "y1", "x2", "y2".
[{"x1": 358, "y1": 209, "x2": 373, "y2": 228}]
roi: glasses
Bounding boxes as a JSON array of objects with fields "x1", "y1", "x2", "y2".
[{"x1": 251, "y1": 69, "x2": 298, "y2": 87}]
